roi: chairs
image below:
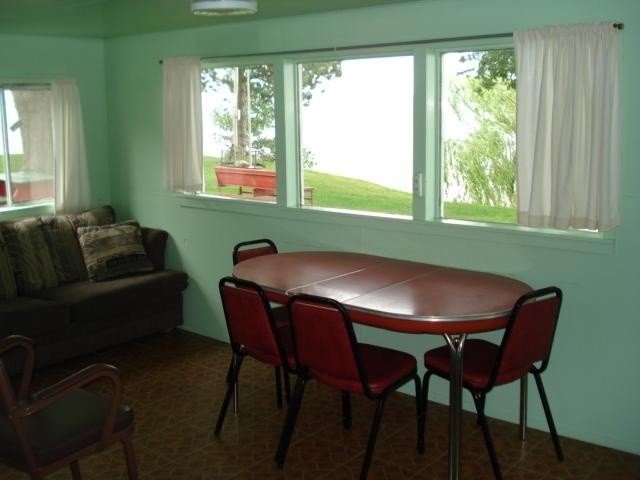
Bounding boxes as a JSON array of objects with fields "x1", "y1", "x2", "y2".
[
  {"x1": 1, "y1": 333, "x2": 140, "y2": 478},
  {"x1": 216, "y1": 276, "x2": 353, "y2": 445},
  {"x1": 272, "y1": 292, "x2": 422, "y2": 478},
  {"x1": 234, "y1": 240, "x2": 278, "y2": 264},
  {"x1": 421, "y1": 286, "x2": 566, "y2": 479}
]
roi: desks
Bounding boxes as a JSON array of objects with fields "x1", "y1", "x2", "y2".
[
  {"x1": 215, "y1": 167, "x2": 276, "y2": 200},
  {"x1": 229, "y1": 250, "x2": 537, "y2": 477}
]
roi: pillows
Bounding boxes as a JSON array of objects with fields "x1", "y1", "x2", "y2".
[{"x1": 76, "y1": 219, "x2": 154, "y2": 283}]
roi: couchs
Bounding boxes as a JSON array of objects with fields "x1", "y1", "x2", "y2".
[{"x1": 0, "y1": 205, "x2": 189, "y2": 376}]
